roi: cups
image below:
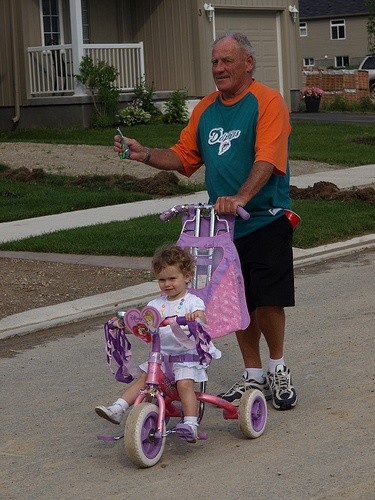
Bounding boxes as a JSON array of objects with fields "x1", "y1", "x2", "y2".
[{"x1": 284, "y1": 209, "x2": 302, "y2": 229}]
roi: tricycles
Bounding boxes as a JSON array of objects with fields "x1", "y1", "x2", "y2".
[{"x1": 96, "y1": 204, "x2": 270, "y2": 468}]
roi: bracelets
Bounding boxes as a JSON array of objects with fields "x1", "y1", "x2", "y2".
[{"x1": 142, "y1": 147, "x2": 151, "y2": 164}]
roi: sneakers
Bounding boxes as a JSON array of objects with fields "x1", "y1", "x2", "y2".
[
  {"x1": 216, "y1": 370, "x2": 271, "y2": 406},
  {"x1": 267, "y1": 363, "x2": 297, "y2": 410}
]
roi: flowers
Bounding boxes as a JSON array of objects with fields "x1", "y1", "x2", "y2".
[{"x1": 303, "y1": 87, "x2": 324, "y2": 98}]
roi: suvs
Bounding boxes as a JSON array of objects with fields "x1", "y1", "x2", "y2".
[{"x1": 358, "y1": 54, "x2": 375, "y2": 98}]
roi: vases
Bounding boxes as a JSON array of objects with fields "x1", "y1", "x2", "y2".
[{"x1": 305, "y1": 96, "x2": 322, "y2": 112}]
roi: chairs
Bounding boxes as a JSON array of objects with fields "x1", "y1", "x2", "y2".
[{"x1": 47, "y1": 39, "x2": 74, "y2": 96}]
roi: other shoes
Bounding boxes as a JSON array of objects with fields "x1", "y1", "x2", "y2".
[
  {"x1": 187, "y1": 423, "x2": 199, "y2": 443},
  {"x1": 95, "y1": 405, "x2": 123, "y2": 425}
]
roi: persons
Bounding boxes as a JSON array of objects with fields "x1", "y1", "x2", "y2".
[
  {"x1": 95, "y1": 244, "x2": 222, "y2": 445},
  {"x1": 114, "y1": 31, "x2": 298, "y2": 411}
]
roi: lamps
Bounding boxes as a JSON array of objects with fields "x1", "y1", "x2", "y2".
[
  {"x1": 204, "y1": 3, "x2": 215, "y2": 21},
  {"x1": 289, "y1": 4, "x2": 299, "y2": 23}
]
